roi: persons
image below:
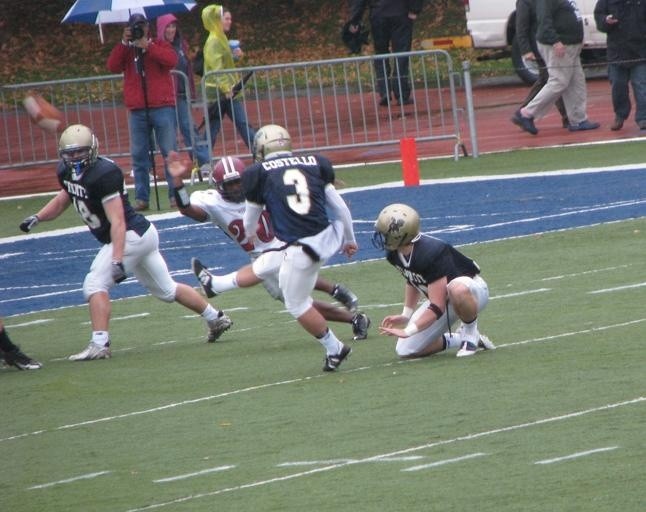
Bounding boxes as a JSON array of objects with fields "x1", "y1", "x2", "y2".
[
  {"x1": 164, "y1": 147, "x2": 372, "y2": 341},
  {"x1": 156, "y1": 13, "x2": 211, "y2": 166},
  {"x1": 202, "y1": 4, "x2": 255, "y2": 151},
  {"x1": 0, "y1": 314, "x2": 41, "y2": 372},
  {"x1": 516, "y1": 0, "x2": 568, "y2": 129},
  {"x1": 374, "y1": 203, "x2": 495, "y2": 357},
  {"x1": 191, "y1": 124, "x2": 356, "y2": 372},
  {"x1": 18, "y1": 122, "x2": 235, "y2": 363},
  {"x1": 594, "y1": 0, "x2": 645, "y2": 131},
  {"x1": 349, "y1": 1, "x2": 425, "y2": 106},
  {"x1": 512, "y1": 0, "x2": 601, "y2": 135},
  {"x1": 105, "y1": 13, "x2": 179, "y2": 212}
]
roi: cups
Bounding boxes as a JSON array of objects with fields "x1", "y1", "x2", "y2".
[{"x1": 228, "y1": 40, "x2": 240, "y2": 62}]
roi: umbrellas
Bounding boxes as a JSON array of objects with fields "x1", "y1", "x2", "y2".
[
  {"x1": 196, "y1": 69, "x2": 253, "y2": 134},
  {"x1": 62, "y1": 0, "x2": 199, "y2": 28},
  {"x1": 340, "y1": 16, "x2": 369, "y2": 54}
]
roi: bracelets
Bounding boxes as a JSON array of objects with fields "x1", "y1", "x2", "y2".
[
  {"x1": 405, "y1": 319, "x2": 419, "y2": 338},
  {"x1": 401, "y1": 305, "x2": 413, "y2": 320},
  {"x1": 173, "y1": 184, "x2": 191, "y2": 209}
]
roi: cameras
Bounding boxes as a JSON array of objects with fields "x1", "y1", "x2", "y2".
[{"x1": 127, "y1": 25, "x2": 144, "y2": 42}]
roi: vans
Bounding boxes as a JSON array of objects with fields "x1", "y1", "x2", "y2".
[{"x1": 464, "y1": 0, "x2": 609, "y2": 87}]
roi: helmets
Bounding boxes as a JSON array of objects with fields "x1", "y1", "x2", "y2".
[
  {"x1": 253, "y1": 125, "x2": 292, "y2": 162},
  {"x1": 59, "y1": 124, "x2": 98, "y2": 174},
  {"x1": 212, "y1": 157, "x2": 246, "y2": 204},
  {"x1": 371, "y1": 204, "x2": 420, "y2": 250}
]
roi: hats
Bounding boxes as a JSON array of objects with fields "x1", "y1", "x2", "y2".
[{"x1": 129, "y1": 13, "x2": 146, "y2": 29}]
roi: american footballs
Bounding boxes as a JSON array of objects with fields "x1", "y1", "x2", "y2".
[{"x1": 22, "y1": 90, "x2": 65, "y2": 135}]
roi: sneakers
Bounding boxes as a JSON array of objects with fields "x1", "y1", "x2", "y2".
[
  {"x1": 133, "y1": 200, "x2": 149, "y2": 211},
  {"x1": 69, "y1": 342, "x2": 110, "y2": 361},
  {"x1": 191, "y1": 256, "x2": 221, "y2": 297},
  {"x1": 397, "y1": 98, "x2": 412, "y2": 106},
  {"x1": 323, "y1": 340, "x2": 351, "y2": 372},
  {"x1": 330, "y1": 286, "x2": 358, "y2": 311},
  {"x1": 510, "y1": 106, "x2": 537, "y2": 133},
  {"x1": 612, "y1": 114, "x2": 628, "y2": 129},
  {"x1": 207, "y1": 312, "x2": 233, "y2": 344},
  {"x1": 379, "y1": 95, "x2": 390, "y2": 109},
  {"x1": 567, "y1": 122, "x2": 600, "y2": 131},
  {"x1": 457, "y1": 332, "x2": 493, "y2": 357},
  {"x1": 0, "y1": 345, "x2": 41, "y2": 370},
  {"x1": 350, "y1": 312, "x2": 370, "y2": 340}
]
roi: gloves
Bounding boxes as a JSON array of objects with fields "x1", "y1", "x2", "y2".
[
  {"x1": 111, "y1": 262, "x2": 126, "y2": 283},
  {"x1": 20, "y1": 216, "x2": 38, "y2": 232}
]
